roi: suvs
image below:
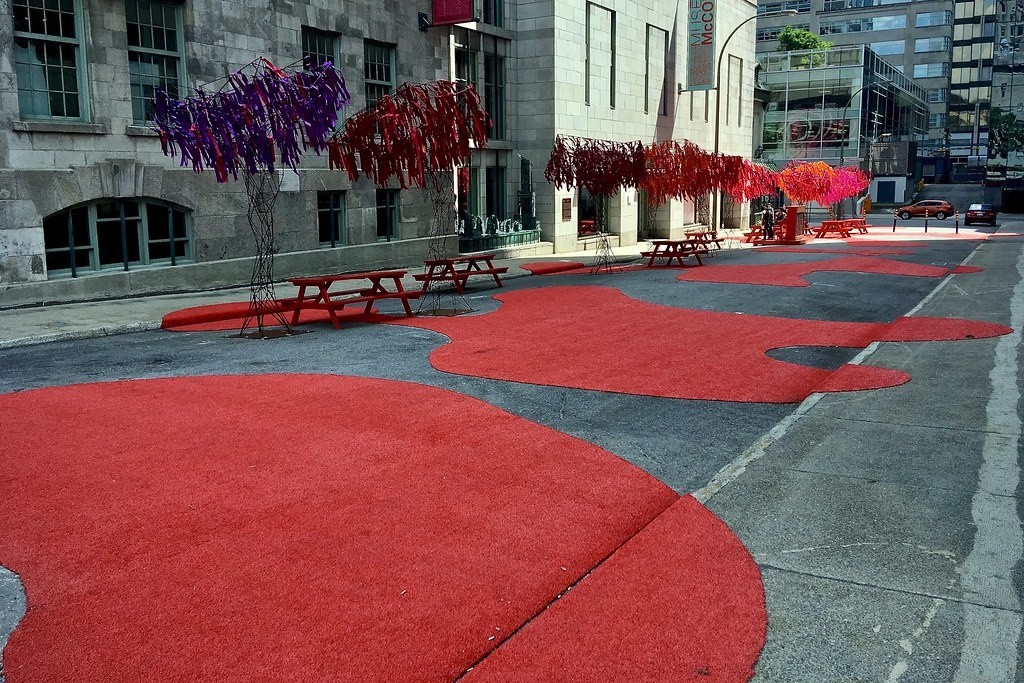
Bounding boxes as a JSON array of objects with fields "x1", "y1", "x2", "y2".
[{"x1": 897, "y1": 199, "x2": 955, "y2": 221}]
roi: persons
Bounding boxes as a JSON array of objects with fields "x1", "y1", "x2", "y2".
[{"x1": 754, "y1": 203, "x2": 788, "y2": 240}]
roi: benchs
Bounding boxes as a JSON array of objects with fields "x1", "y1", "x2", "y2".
[
  {"x1": 684, "y1": 237, "x2": 726, "y2": 245},
  {"x1": 413, "y1": 267, "x2": 509, "y2": 281},
  {"x1": 812, "y1": 224, "x2": 872, "y2": 232},
  {"x1": 743, "y1": 232, "x2": 787, "y2": 236},
  {"x1": 635, "y1": 250, "x2": 709, "y2": 257},
  {"x1": 277, "y1": 288, "x2": 426, "y2": 310}
]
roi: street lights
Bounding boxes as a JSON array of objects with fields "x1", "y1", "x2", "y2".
[
  {"x1": 836, "y1": 79, "x2": 895, "y2": 219},
  {"x1": 711, "y1": 9, "x2": 799, "y2": 236}
]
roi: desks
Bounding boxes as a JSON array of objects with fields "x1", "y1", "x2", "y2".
[
  {"x1": 422, "y1": 254, "x2": 503, "y2": 295},
  {"x1": 815, "y1": 219, "x2": 868, "y2": 238},
  {"x1": 745, "y1": 225, "x2": 780, "y2": 243},
  {"x1": 292, "y1": 269, "x2": 413, "y2": 328},
  {"x1": 646, "y1": 230, "x2": 721, "y2": 268}
]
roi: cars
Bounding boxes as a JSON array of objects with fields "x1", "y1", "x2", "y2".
[{"x1": 963, "y1": 203, "x2": 997, "y2": 226}]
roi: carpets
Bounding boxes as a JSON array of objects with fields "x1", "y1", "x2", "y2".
[
  {"x1": 519, "y1": 254, "x2": 986, "y2": 287},
  {"x1": 0, "y1": 372, "x2": 768, "y2": 683},
  {"x1": 727, "y1": 232, "x2": 928, "y2": 248},
  {"x1": 752, "y1": 246, "x2": 913, "y2": 255},
  {"x1": 160, "y1": 280, "x2": 1014, "y2": 402}
]
